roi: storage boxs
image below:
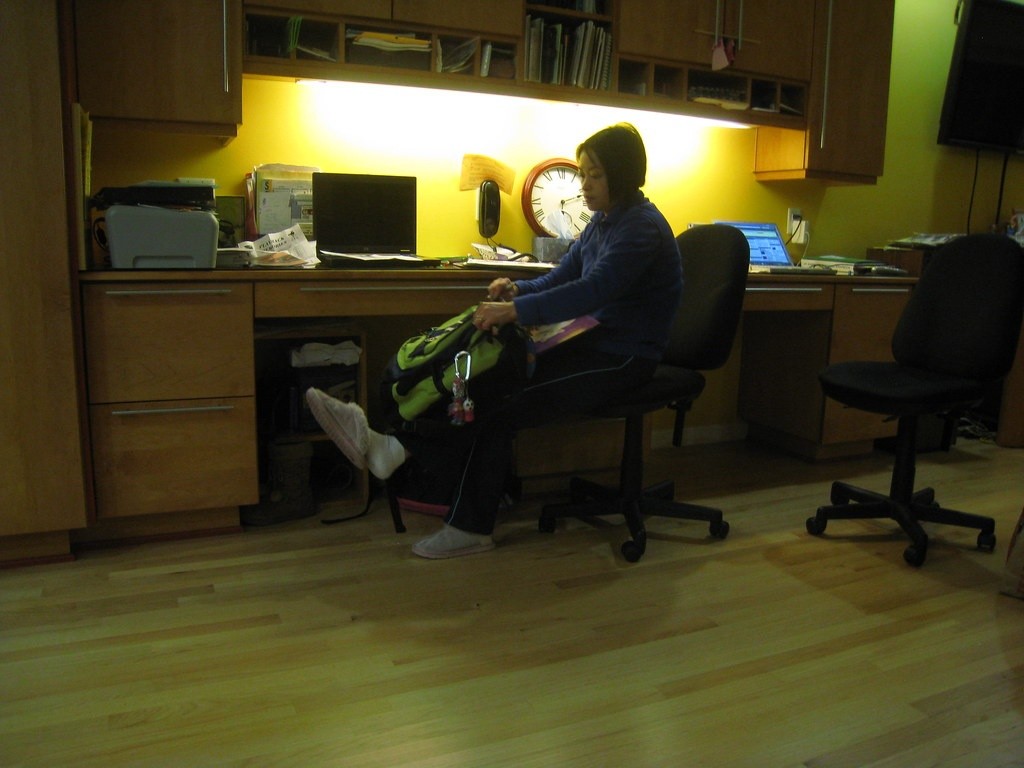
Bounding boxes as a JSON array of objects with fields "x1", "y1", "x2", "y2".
[{"x1": 867, "y1": 244, "x2": 945, "y2": 277}]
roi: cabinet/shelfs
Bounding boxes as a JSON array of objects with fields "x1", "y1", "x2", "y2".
[{"x1": 1, "y1": 1, "x2": 1023, "y2": 568}]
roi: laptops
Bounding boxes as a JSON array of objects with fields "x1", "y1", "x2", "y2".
[
  {"x1": 311, "y1": 173, "x2": 441, "y2": 269},
  {"x1": 716, "y1": 221, "x2": 838, "y2": 275}
]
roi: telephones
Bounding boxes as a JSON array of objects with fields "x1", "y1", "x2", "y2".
[{"x1": 479, "y1": 179, "x2": 502, "y2": 237}]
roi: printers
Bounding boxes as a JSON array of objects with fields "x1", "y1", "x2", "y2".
[{"x1": 105, "y1": 205, "x2": 220, "y2": 270}]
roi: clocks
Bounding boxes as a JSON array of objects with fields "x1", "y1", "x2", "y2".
[{"x1": 521, "y1": 157, "x2": 595, "y2": 239}]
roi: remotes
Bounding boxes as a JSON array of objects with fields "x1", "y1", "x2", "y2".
[{"x1": 872, "y1": 266, "x2": 908, "y2": 277}]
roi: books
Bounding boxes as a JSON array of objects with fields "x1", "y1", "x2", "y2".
[
  {"x1": 895, "y1": 230, "x2": 965, "y2": 247},
  {"x1": 801, "y1": 255, "x2": 901, "y2": 275},
  {"x1": 247, "y1": 0, "x2": 616, "y2": 92},
  {"x1": 526, "y1": 312, "x2": 600, "y2": 355},
  {"x1": 246, "y1": 162, "x2": 313, "y2": 235},
  {"x1": 749, "y1": 264, "x2": 838, "y2": 275}
]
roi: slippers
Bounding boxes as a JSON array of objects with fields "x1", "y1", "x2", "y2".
[
  {"x1": 411, "y1": 523, "x2": 497, "y2": 558},
  {"x1": 305, "y1": 386, "x2": 373, "y2": 470}
]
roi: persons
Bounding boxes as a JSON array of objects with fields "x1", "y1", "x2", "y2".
[{"x1": 305, "y1": 125, "x2": 686, "y2": 559}]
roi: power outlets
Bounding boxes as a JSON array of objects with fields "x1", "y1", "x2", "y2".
[{"x1": 787, "y1": 207, "x2": 801, "y2": 235}]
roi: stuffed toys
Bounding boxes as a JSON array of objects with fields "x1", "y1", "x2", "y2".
[{"x1": 447, "y1": 377, "x2": 465, "y2": 423}]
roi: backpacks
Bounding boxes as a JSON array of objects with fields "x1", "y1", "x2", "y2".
[{"x1": 373, "y1": 296, "x2": 539, "y2": 424}]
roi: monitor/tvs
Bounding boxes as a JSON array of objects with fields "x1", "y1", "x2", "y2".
[{"x1": 937, "y1": 0, "x2": 1024, "y2": 158}]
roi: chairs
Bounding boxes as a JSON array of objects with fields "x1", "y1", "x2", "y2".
[
  {"x1": 805, "y1": 231, "x2": 1023, "y2": 566},
  {"x1": 538, "y1": 224, "x2": 749, "y2": 564}
]
